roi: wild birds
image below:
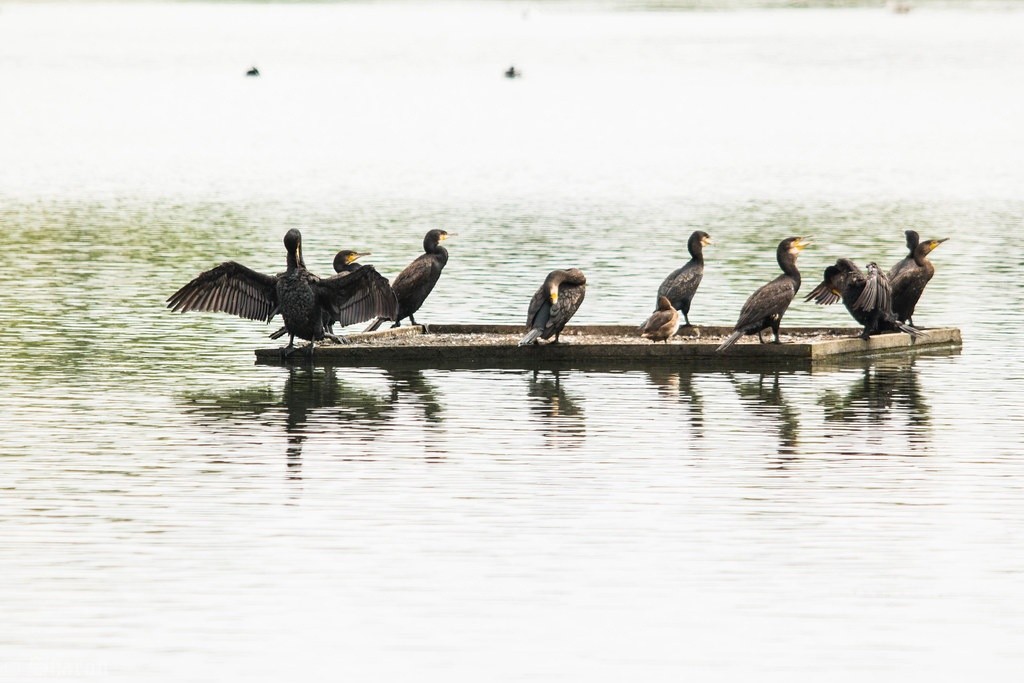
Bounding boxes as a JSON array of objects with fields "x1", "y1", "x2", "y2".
[
  {"x1": 652, "y1": 230, "x2": 715, "y2": 327},
  {"x1": 164, "y1": 225, "x2": 459, "y2": 360},
  {"x1": 504, "y1": 66, "x2": 522, "y2": 79},
  {"x1": 247, "y1": 66, "x2": 261, "y2": 76},
  {"x1": 517, "y1": 268, "x2": 587, "y2": 349},
  {"x1": 802, "y1": 230, "x2": 951, "y2": 340},
  {"x1": 714, "y1": 234, "x2": 817, "y2": 354},
  {"x1": 641, "y1": 294, "x2": 680, "y2": 344}
]
